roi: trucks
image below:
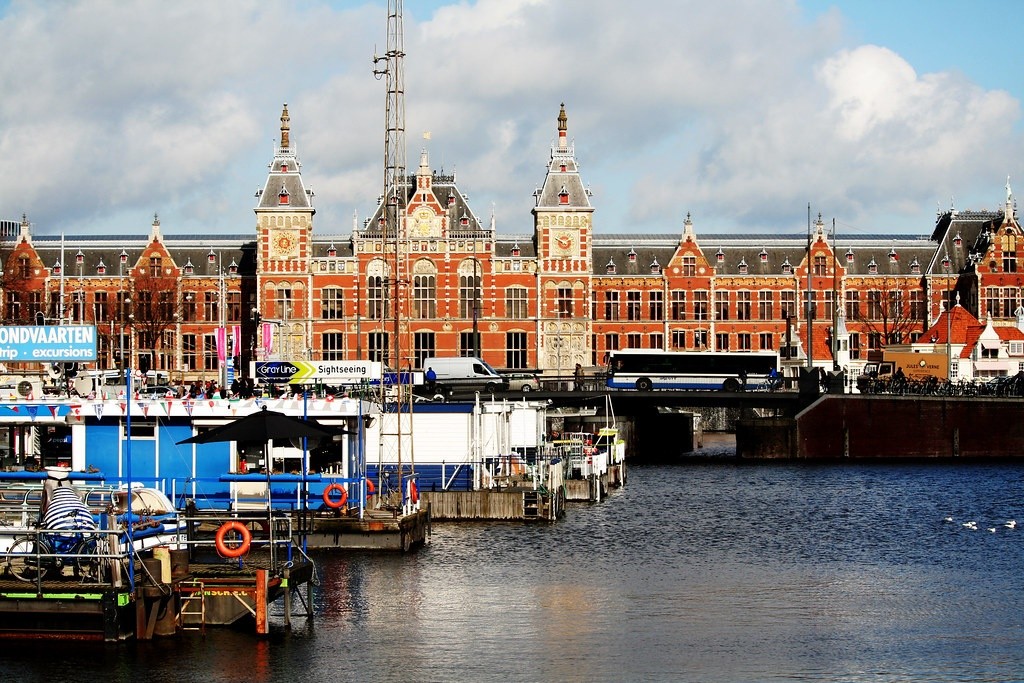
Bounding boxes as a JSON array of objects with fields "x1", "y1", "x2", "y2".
[{"x1": 855, "y1": 348, "x2": 948, "y2": 394}]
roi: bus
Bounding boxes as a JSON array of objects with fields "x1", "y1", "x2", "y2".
[{"x1": 602, "y1": 347, "x2": 782, "y2": 393}]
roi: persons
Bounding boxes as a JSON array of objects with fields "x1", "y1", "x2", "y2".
[
  {"x1": 189, "y1": 378, "x2": 249, "y2": 399},
  {"x1": 573, "y1": 363, "x2": 587, "y2": 392},
  {"x1": 426, "y1": 367, "x2": 437, "y2": 395},
  {"x1": 737, "y1": 363, "x2": 907, "y2": 396}
]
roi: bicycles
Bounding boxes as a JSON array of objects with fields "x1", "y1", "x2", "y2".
[
  {"x1": 865, "y1": 369, "x2": 1024, "y2": 398},
  {"x1": 5, "y1": 507, "x2": 107, "y2": 582},
  {"x1": 756, "y1": 377, "x2": 787, "y2": 393}
]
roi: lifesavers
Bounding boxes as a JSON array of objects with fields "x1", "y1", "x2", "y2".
[
  {"x1": 366, "y1": 481, "x2": 374, "y2": 501},
  {"x1": 411, "y1": 481, "x2": 420, "y2": 504},
  {"x1": 325, "y1": 486, "x2": 348, "y2": 510},
  {"x1": 216, "y1": 520, "x2": 251, "y2": 560}
]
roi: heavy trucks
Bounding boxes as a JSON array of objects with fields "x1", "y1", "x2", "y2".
[{"x1": 249, "y1": 359, "x2": 404, "y2": 395}]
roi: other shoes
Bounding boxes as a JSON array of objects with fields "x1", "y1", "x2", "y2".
[{"x1": 584, "y1": 386, "x2": 588, "y2": 390}]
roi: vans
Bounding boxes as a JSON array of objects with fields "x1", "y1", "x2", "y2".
[{"x1": 423, "y1": 356, "x2": 504, "y2": 395}]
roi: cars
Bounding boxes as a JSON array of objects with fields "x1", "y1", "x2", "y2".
[
  {"x1": 137, "y1": 385, "x2": 179, "y2": 397},
  {"x1": 506, "y1": 373, "x2": 541, "y2": 392}
]
